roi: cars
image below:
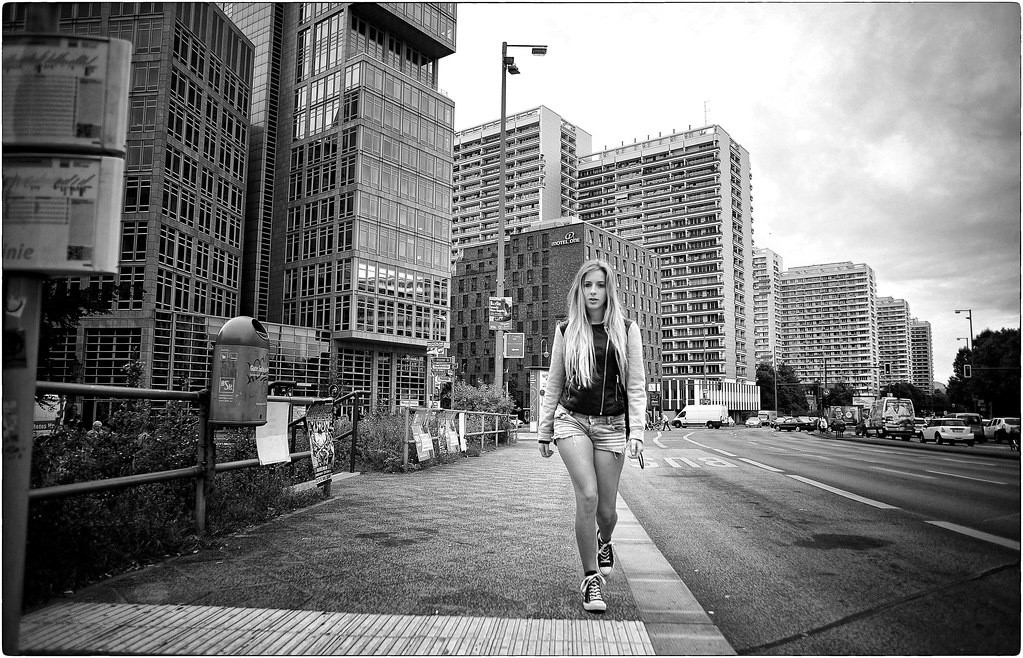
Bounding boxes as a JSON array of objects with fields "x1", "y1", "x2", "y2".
[
  {"x1": 855, "y1": 419, "x2": 867, "y2": 436},
  {"x1": 815, "y1": 417, "x2": 847, "y2": 433},
  {"x1": 913, "y1": 417, "x2": 927, "y2": 436},
  {"x1": 983, "y1": 417, "x2": 1021, "y2": 443},
  {"x1": 721, "y1": 417, "x2": 735, "y2": 427},
  {"x1": 770, "y1": 417, "x2": 794, "y2": 428},
  {"x1": 777, "y1": 417, "x2": 815, "y2": 432},
  {"x1": 745, "y1": 417, "x2": 763, "y2": 428},
  {"x1": 919, "y1": 417, "x2": 975, "y2": 447}
]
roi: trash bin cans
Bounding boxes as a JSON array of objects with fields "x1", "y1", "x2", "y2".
[{"x1": 206, "y1": 316, "x2": 270, "y2": 426}]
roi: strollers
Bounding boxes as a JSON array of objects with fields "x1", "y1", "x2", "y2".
[{"x1": 648, "y1": 419, "x2": 661, "y2": 431}]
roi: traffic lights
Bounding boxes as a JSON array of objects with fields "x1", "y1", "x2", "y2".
[
  {"x1": 885, "y1": 364, "x2": 891, "y2": 375},
  {"x1": 964, "y1": 365, "x2": 971, "y2": 378}
]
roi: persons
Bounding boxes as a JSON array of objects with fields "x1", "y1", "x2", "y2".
[
  {"x1": 645, "y1": 411, "x2": 661, "y2": 431},
  {"x1": 661, "y1": 413, "x2": 671, "y2": 431},
  {"x1": 538, "y1": 259, "x2": 648, "y2": 612},
  {"x1": 818, "y1": 417, "x2": 828, "y2": 432}
]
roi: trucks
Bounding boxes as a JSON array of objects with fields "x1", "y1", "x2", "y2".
[
  {"x1": 827, "y1": 406, "x2": 859, "y2": 426},
  {"x1": 758, "y1": 411, "x2": 777, "y2": 426}
]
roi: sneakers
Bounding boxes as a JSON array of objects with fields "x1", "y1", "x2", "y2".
[
  {"x1": 580, "y1": 573, "x2": 606, "y2": 611},
  {"x1": 596, "y1": 528, "x2": 614, "y2": 576}
]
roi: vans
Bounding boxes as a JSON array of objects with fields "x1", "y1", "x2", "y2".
[
  {"x1": 672, "y1": 404, "x2": 729, "y2": 429},
  {"x1": 943, "y1": 413, "x2": 985, "y2": 443},
  {"x1": 865, "y1": 393, "x2": 916, "y2": 441}
]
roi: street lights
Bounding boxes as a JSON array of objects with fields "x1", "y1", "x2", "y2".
[
  {"x1": 492, "y1": 44, "x2": 548, "y2": 392},
  {"x1": 824, "y1": 358, "x2": 832, "y2": 418},
  {"x1": 703, "y1": 321, "x2": 716, "y2": 404},
  {"x1": 957, "y1": 337, "x2": 968, "y2": 413},
  {"x1": 857, "y1": 379, "x2": 869, "y2": 396},
  {"x1": 541, "y1": 339, "x2": 549, "y2": 366},
  {"x1": 774, "y1": 345, "x2": 780, "y2": 410},
  {"x1": 955, "y1": 309, "x2": 976, "y2": 413}
]
